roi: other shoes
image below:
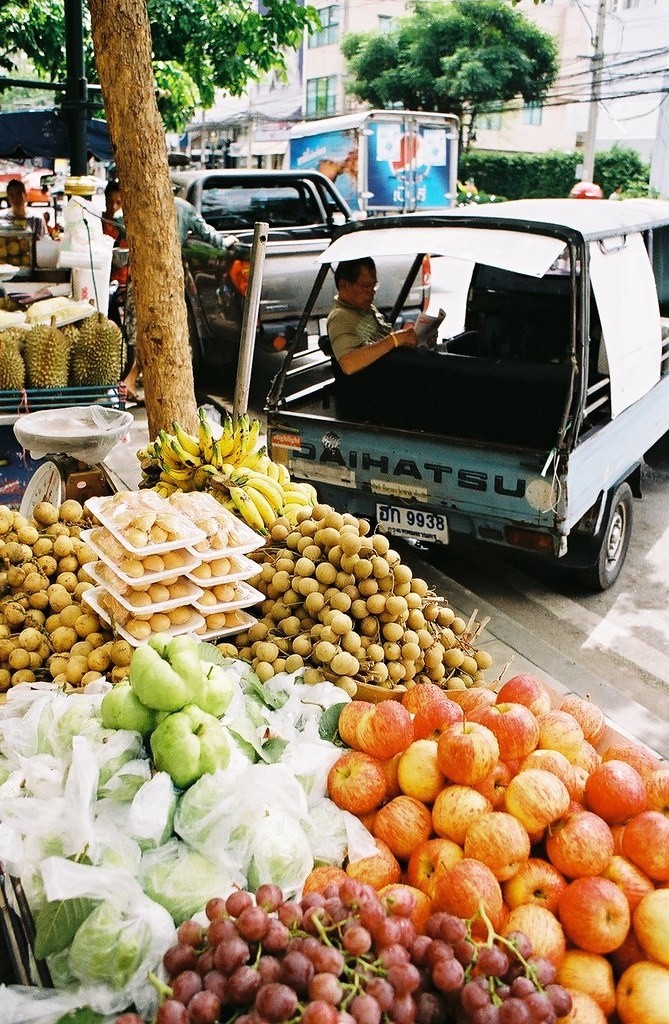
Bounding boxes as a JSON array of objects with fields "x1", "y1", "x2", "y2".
[{"x1": 126, "y1": 391, "x2": 146, "y2": 406}]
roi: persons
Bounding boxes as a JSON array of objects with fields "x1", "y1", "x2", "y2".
[
  {"x1": 114, "y1": 184, "x2": 244, "y2": 397},
  {"x1": 318, "y1": 150, "x2": 357, "y2": 188},
  {"x1": 609, "y1": 186, "x2": 622, "y2": 200},
  {"x1": 101, "y1": 169, "x2": 134, "y2": 382},
  {"x1": 327, "y1": 256, "x2": 438, "y2": 375},
  {"x1": 0, "y1": 178, "x2": 54, "y2": 239}
]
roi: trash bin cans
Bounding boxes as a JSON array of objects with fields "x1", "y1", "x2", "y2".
[{"x1": 569, "y1": 182, "x2": 605, "y2": 199}]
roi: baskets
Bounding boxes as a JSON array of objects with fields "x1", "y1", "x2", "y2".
[{"x1": 322, "y1": 669, "x2": 485, "y2": 705}]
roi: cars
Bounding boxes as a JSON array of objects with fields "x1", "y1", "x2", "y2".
[
  {"x1": 171, "y1": 169, "x2": 432, "y2": 391},
  {"x1": 0, "y1": 160, "x2": 109, "y2": 209}
]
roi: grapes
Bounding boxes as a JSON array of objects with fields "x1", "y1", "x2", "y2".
[{"x1": 116, "y1": 878, "x2": 572, "y2": 1024}]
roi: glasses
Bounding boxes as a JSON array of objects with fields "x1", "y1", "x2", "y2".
[{"x1": 348, "y1": 278, "x2": 380, "y2": 292}]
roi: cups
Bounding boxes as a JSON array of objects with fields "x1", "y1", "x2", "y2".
[
  {"x1": 111, "y1": 248, "x2": 129, "y2": 268},
  {"x1": 37, "y1": 241, "x2": 60, "y2": 269}
]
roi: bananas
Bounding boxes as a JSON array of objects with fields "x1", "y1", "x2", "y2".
[{"x1": 139, "y1": 408, "x2": 318, "y2": 534}]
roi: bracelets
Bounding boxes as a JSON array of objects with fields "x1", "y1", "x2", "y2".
[{"x1": 390, "y1": 331, "x2": 398, "y2": 347}]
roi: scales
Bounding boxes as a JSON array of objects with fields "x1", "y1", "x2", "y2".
[{"x1": 13, "y1": 406, "x2": 135, "y2": 532}]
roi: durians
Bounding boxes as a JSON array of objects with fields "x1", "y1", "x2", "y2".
[{"x1": 0, "y1": 312, "x2": 125, "y2": 392}]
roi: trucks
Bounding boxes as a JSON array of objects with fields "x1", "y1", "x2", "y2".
[{"x1": 281, "y1": 108, "x2": 463, "y2": 216}]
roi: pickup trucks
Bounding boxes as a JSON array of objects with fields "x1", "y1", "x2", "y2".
[{"x1": 264, "y1": 195, "x2": 669, "y2": 593}]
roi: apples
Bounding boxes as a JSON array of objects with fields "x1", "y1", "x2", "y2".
[{"x1": 304, "y1": 675, "x2": 669, "y2": 1024}]
